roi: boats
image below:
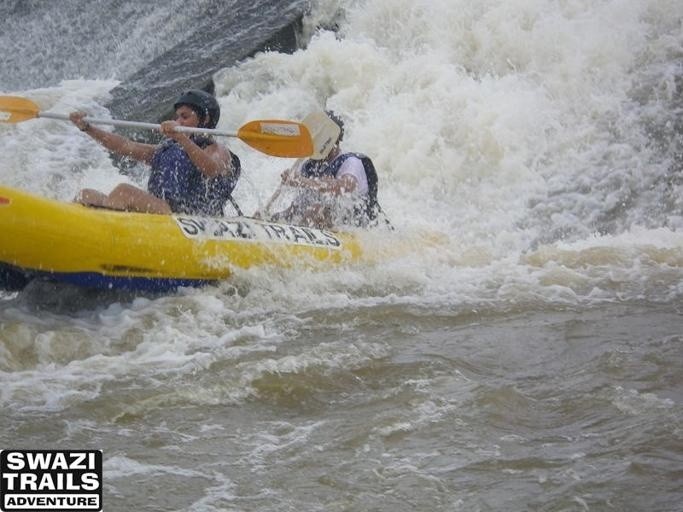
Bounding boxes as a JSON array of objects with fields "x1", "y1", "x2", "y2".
[{"x1": 2, "y1": 185, "x2": 441, "y2": 294}]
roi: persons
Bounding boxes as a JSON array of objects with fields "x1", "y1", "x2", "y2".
[
  {"x1": 255, "y1": 109, "x2": 371, "y2": 230},
  {"x1": 70, "y1": 87, "x2": 242, "y2": 216}
]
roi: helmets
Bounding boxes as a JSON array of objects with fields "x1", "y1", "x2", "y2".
[
  {"x1": 174, "y1": 89, "x2": 220, "y2": 130},
  {"x1": 323, "y1": 110, "x2": 344, "y2": 141}
]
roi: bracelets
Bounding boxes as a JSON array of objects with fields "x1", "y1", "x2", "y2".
[{"x1": 77, "y1": 120, "x2": 89, "y2": 134}]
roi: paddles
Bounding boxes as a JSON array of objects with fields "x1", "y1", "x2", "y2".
[
  {"x1": 0, "y1": 97, "x2": 313, "y2": 158},
  {"x1": 254, "y1": 104, "x2": 342, "y2": 219}
]
